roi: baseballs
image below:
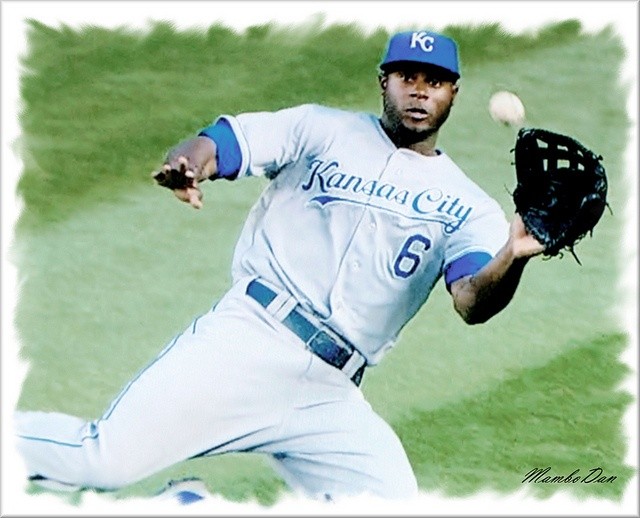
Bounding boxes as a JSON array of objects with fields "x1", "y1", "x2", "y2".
[{"x1": 490, "y1": 91, "x2": 525, "y2": 126}]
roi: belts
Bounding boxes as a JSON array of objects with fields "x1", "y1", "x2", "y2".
[{"x1": 246, "y1": 281, "x2": 365, "y2": 387}]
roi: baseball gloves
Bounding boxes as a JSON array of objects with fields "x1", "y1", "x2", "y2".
[{"x1": 513, "y1": 129, "x2": 608, "y2": 256}]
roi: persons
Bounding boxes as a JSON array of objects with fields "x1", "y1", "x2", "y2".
[{"x1": 18, "y1": 30, "x2": 547, "y2": 509}]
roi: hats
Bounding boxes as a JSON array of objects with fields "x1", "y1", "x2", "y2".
[{"x1": 379, "y1": 31, "x2": 459, "y2": 77}]
